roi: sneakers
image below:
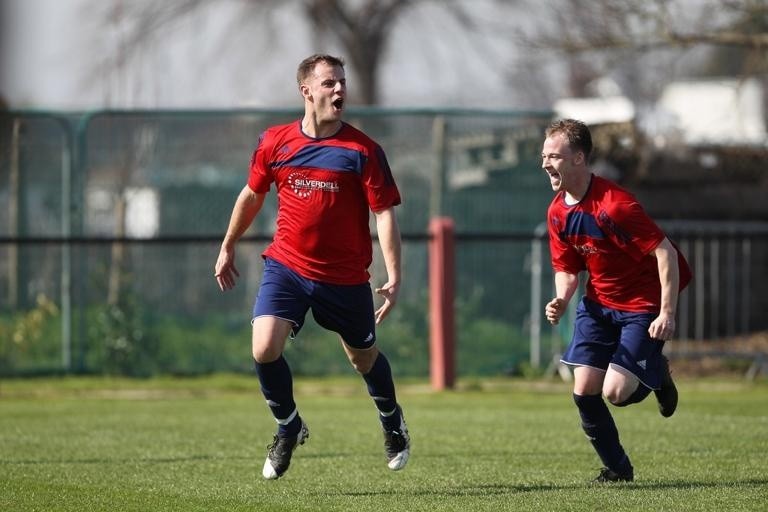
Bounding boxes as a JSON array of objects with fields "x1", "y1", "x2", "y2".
[
  {"x1": 592, "y1": 457, "x2": 634, "y2": 484},
  {"x1": 376, "y1": 403, "x2": 411, "y2": 472},
  {"x1": 261, "y1": 417, "x2": 309, "y2": 481},
  {"x1": 653, "y1": 356, "x2": 680, "y2": 419}
]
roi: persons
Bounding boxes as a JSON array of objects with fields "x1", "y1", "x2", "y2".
[
  {"x1": 212, "y1": 55, "x2": 411, "y2": 481},
  {"x1": 540, "y1": 119, "x2": 692, "y2": 483}
]
